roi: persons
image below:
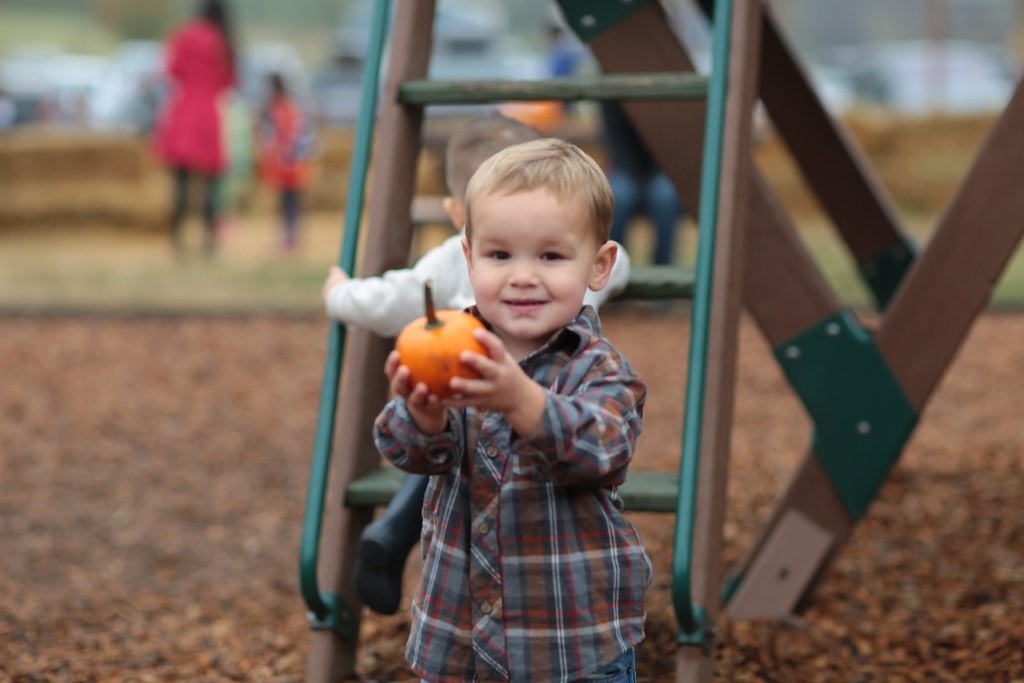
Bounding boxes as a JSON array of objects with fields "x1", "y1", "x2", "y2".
[
  {"x1": 157, "y1": 1, "x2": 236, "y2": 263},
  {"x1": 546, "y1": 27, "x2": 584, "y2": 113},
  {"x1": 375, "y1": 138, "x2": 651, "y2": 683},
  {"x1": 602, "y1": 99, "x2": 680, "y2": 265},
  {"x1": 253, "y1": 72, "x2": 306, "y2": 246},
  {"x1": 322, "y1": 116, "x2": 631, "y2": 616}
]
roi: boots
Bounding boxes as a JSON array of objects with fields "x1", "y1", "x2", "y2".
[{"x1": 353, "y1": 474, "x2": 427, "y2": 616}]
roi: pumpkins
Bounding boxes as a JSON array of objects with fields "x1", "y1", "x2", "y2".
[{"x1": 394, "y1": 280, "x2": 491, "y2": 408}]
